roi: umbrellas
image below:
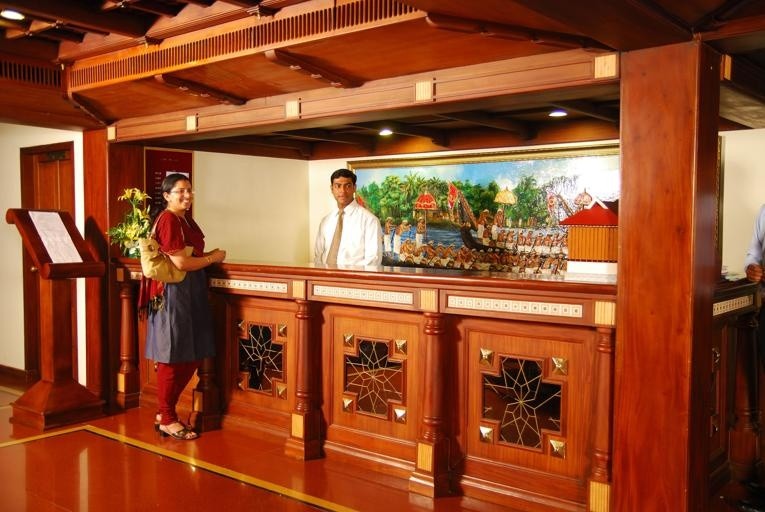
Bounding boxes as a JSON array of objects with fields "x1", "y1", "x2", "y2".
[
  {"x1": 491, "y1": 188, "x2": 518, "y2": 225},
  {"x1": 574, "y1": 188, "x2": 592, "y2": 210},
  {"x1": 413, "y1": 194, "x2": 438, "y2": 242}
]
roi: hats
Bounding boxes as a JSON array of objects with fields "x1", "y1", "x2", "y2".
[{"x1": 386, "y1": 208, "x2": 568, "y2": 259}]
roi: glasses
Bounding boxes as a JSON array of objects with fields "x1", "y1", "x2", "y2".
[{"x1": 169, "y1": 190, "x2": 192, "y2": 194}]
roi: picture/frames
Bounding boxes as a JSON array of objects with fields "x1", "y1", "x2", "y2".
[{"x1": 346, "y1": 142, "x2": 619, "y2": 275}]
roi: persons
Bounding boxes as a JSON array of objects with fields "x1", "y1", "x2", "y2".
[
  {"x1": 742, "y1": 203, "x2": 765, "y2": 283},
  {"x1": 315, "y1": 169, "x2": 383, "y2": 265},
  {"x1": 137, "y1": 171, "x2": 228, "y2": 443},
  {"x1": 382, "y1": 208, "x2": 569, "y2": 276}
]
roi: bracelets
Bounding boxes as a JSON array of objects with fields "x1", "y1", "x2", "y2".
[{"x1": 206, "y1": 255, "x2": 212, "y2": 266}]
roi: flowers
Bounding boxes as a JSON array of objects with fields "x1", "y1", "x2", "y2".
[{"x1": 105, "y1": 188, "x2": 154, "y2": 256}]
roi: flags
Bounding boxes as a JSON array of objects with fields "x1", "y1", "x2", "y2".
[{"x1": 447, "y1": 183, "x2": 458, "y2": 211}]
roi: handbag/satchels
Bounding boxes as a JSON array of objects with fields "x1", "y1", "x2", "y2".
[{"x1": 139, "y1": 209, "x2": 194, "y2": 283}]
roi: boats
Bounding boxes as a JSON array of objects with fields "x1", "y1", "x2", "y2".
[
  {"x1": 381, "y1": 255, "x2": 561, "y2": 275},
  {"x1": 448, "y1": 185, "x2": 569, "y2": 258}
]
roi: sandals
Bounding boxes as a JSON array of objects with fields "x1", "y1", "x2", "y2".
[
  {"x1": 155, "y1": 419, "x2": 198, "y2": 439},
  {"x1": 326, "y1": 210, "x2": 344, "y2": 269}
]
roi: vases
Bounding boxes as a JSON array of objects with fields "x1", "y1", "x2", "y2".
[{"x1": 129, "y1": 248, "x2": 140, "y2": 259}]
